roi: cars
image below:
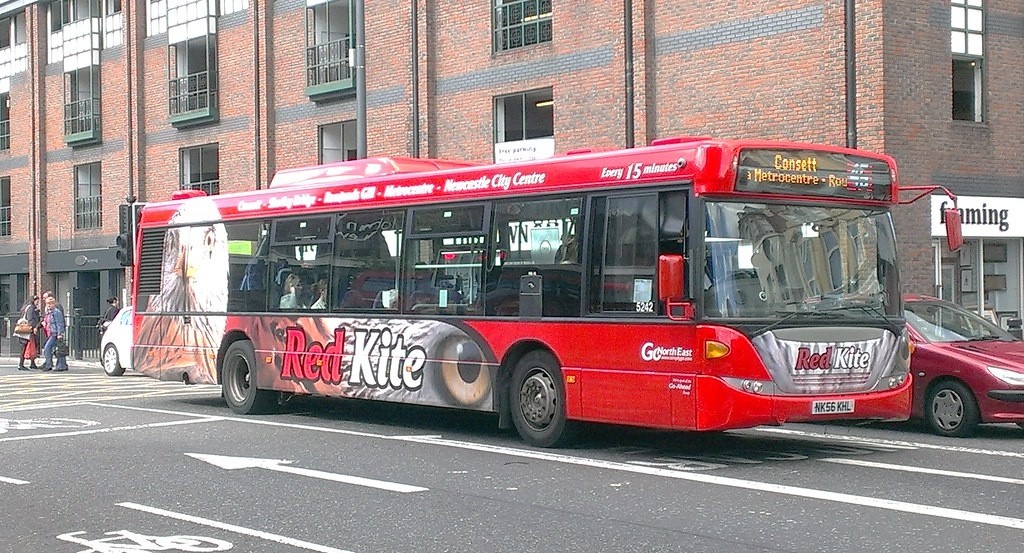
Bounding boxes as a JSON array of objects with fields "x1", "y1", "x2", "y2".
[
  {"x1": 99, "y1": 306, "x2": 132, "y2": 376},
  {"x1": 901, "y1": 292, "x2": 1024, "y2": 439}
]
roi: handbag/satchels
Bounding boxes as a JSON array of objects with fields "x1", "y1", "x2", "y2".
[
  {"x1": 99, "y1": 307, "x2": 113, "y2": 330},
  {"x1": 24, "y1": 334, "x2": 37, "y2": 359},
  {"x1": 12, "y1": 304, "x2": 32, "y2": 340},
  {"x1": 56, "y1": 339, "x2": 69, "y2": 357}
]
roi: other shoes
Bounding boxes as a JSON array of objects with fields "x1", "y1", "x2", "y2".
[
  {"x1": 30, "y1": 363, "x2": 38, "y2": 369},
  {"x1": 43, "y1": 366, "x2": 52, "y2": 372},
  {"x1": 18, "y1": 366, "x2": 30, "y2": 371},
  {"x1": 53, "y1": 367, "x2": 68, "y2": 371}
]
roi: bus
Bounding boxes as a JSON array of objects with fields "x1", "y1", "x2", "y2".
[{"x1": 129, "y1": 134, "x2": 963, "y2": 449}]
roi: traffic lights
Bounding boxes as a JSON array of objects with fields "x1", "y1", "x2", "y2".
[{"x1": 114, "y1": 232, "x2": 133, "y2": 267}]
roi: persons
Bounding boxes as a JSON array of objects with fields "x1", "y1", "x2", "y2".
[
  {"x1": 96, "y1": 297, "x2": 123, "y2": 335},
  {"x1": 278, "y1": 271, "x2": 329, "y2": 309},
  {"x1": 17, "y1": 291, "x2": 68, "y2": 371}
]
